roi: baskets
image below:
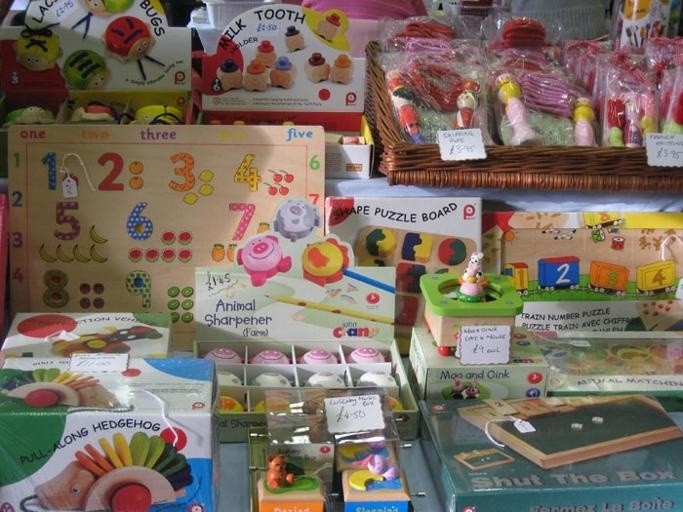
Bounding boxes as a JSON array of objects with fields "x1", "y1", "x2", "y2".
[{"x1": 364, "y1": 34, "x2": 683, "y2": 198}]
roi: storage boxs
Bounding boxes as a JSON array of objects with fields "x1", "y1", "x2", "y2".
[
  {"x1": 2, "y1": 311, "x2": 173, "y2": 359},
  {"x1": 419, "y1": 396, "x2": 683, "y2": 511},
  {"x1": 0, "y1": 355, "x2": 223, "y2": 512}
]
